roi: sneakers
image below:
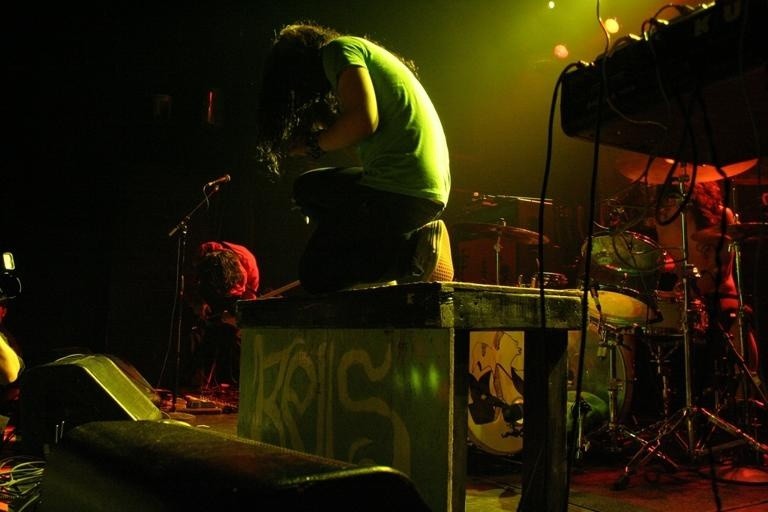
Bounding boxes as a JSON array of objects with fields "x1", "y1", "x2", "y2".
[{"x1": 408, "y1": 219, "x2": 455, "y2": 283}]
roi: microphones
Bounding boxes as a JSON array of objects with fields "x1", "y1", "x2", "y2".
[{"x1": 208, "y1": 174, "x2": 231, "y2": 187}]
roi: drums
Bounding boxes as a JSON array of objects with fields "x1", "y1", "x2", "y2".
[
  {"x1": 641, "y1": 296, "x2": 708, "y2": 337},
  {"x1": 466, "y1": 320, "x2": 638, "y2": 457},
  {"x1": 457, "y1": 232, "x2": 561, "y2": 287},
  {"x1": 575, "y1": 229, "x2": 667, "y2": 329}
]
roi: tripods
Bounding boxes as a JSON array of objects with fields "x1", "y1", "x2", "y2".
[{"x1": 570, "y1": 162, "x2": 767, "y2": 511}]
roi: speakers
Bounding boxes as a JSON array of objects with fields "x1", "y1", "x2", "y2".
[
  {"x1": 38, "y1": 419, "x2": 432, "y2": 511},
  {"x1": 16, "y1": 352, "x2": 164, "y2": 467},
  {"x1": 106, "y1": 353, "x2": 161, "y2": 407}
]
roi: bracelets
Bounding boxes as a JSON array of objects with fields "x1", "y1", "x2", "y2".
[{"x1": 303, "y1": 130, "x2": 326, "y2": 158}]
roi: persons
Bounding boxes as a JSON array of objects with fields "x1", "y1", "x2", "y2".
[
  {"x1": 254, "y1": 18, "x2": 454, "y2": 294},
  {"x1": 181, "y1": 240, "x2": 260, "y2": 391},
  {"x1": 648, "y1": 180, "x2": 737, "y2": 294}
]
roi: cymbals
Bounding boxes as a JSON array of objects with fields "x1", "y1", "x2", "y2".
[
  {"x1": 614, "y1": 150, "x2": 758, "y2": 186},
  {"x1": 692, "y1": 222, "x2": 766, "y2": 247},
  {"x1": 451, "y1": 222, "x2": 550, "y2": 246}
]
